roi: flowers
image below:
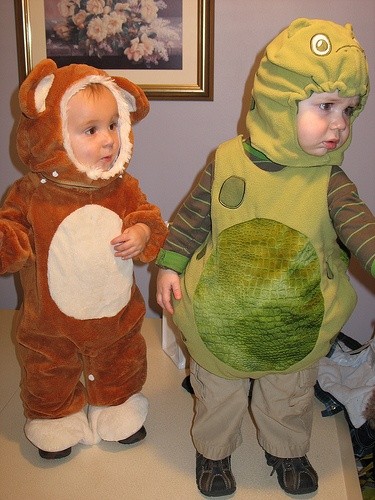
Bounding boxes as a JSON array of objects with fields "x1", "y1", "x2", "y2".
[{"x1": 47, "y1": 0, "x2": 179, "y2": 65}]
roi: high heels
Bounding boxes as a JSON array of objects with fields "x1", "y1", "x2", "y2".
[{"x1": 314, "y1": 379, "x2": 345, "y2": 418}]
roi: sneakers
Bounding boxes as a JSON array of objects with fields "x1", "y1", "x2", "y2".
[
  {"x1": 266, "y1": 452, "x2": 319, "y2": 496},
  {"x1": 195, "y1": 451, "x2": 236, "y2": 497}
]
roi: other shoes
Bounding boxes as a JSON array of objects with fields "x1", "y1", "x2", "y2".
[
  {"x1": 39, "y1": 448, "x2": 72, "y2": 460},
  {"x1": 119, "y1": 425, "x2": 146, "y2": 445}
]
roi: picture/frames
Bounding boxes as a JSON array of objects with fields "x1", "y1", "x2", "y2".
[{"x1": 13, "y1": 0, "x2": 214, "y2": 101}]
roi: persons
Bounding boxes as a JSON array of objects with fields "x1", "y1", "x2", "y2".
[
  {"x1": 154, "y1": 17, "x2": 375, "y2": 500},
  {"x1": 0, "y1": 60, "x2": 171, "y2": 460}
]
